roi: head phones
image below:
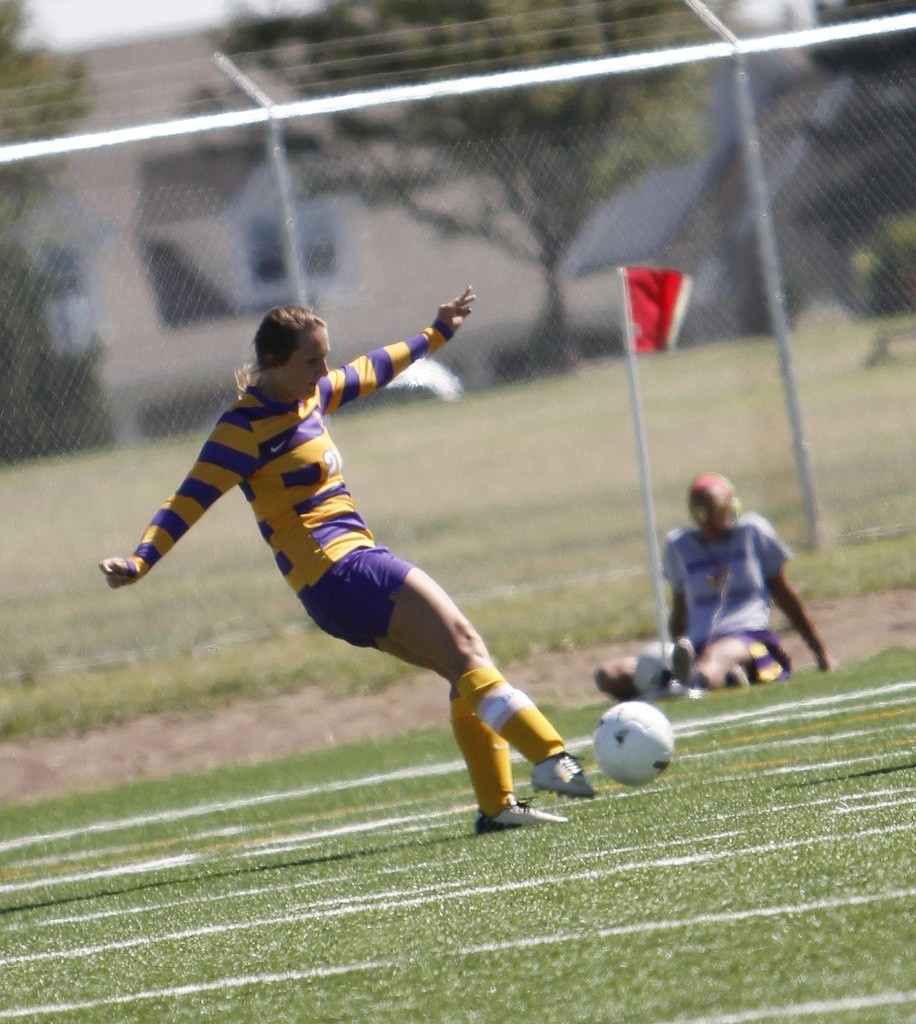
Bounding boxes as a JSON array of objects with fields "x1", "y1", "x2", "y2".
[{"x1": 686, "y1": 476, "x2": 743, "y2": 524}]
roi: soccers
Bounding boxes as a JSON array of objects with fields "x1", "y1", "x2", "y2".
[{"x1": 591, "y1": 699, "x2": 675, "y2": 788}]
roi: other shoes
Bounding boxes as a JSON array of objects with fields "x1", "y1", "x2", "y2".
[
  {"x1": 672, "y1": 637, "x2": 694, "y2": 682},
  {"x1": 725, "y1": 667, "x2": 752, "y2": 689}
]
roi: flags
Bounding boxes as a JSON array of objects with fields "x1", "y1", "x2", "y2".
[{"x1": 622, "y1": 269, "x2": 690, "y2": 352}]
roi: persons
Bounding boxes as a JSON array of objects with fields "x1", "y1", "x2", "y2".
[
  {"x1": 98, "y1": 285, "x2": 588, "y2": 832},
  {"x1": 593, "y1": 470, "x2": 838, "y2": 702}
]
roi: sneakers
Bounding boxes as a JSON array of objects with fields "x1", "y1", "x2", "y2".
[
  {"x1": 530, "y1": 752, "x2": 596, "y2": 799},
  {"x1": 475, "y1": 795, "x2": 569, "y2": 833}
]
